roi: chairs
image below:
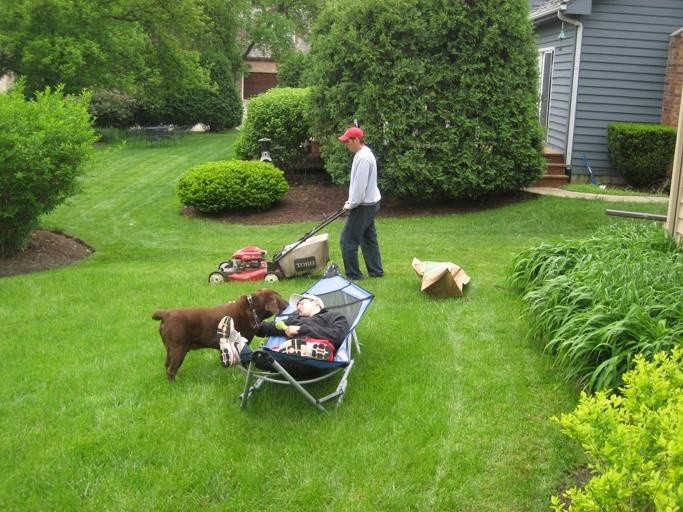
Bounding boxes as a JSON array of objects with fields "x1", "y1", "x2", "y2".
[{"x1": 240, "y1": 263, "x2": 374, "y2": 416}]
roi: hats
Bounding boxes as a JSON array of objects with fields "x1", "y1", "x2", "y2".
[
  {"x1": 339, "y1": 127, "x2": 363, "y2": 141},
  {"x1": 289, "y1": 293, "x2": 325, "y2": 309}
]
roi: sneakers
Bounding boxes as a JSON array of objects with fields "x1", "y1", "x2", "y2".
[
  {"x1": 279, "y1": 339, "x2": 331, "y2": 360},
  {"x1": 218, "y1": 316, "x2": 240, "y2": 367}
]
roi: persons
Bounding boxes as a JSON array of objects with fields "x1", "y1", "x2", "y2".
[
  {"x1": 338, "y1": 126, "x2": 383, "y2": 281},
  {"x1": 217, "y1": 293, "x2": 349, "y2": 368}
]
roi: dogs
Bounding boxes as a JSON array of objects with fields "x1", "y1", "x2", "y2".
[{"x1": 152, "y1": 288, "x2": 289, "y2": 384}]
